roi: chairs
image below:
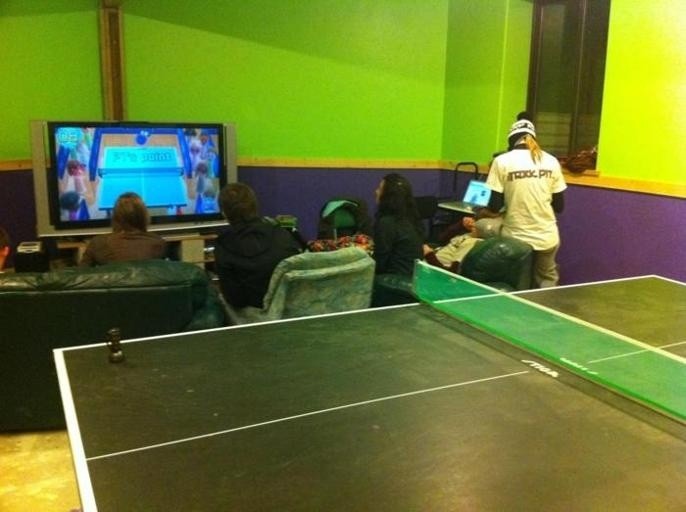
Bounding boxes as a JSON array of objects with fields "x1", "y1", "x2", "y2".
[
  {"x1": 220, "y1": 247, "x2": 376, "y2": 325},
  {"x1": 412, "y1": 196, "x2": 442, "y2": 248}
]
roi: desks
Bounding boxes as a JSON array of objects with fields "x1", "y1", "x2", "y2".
[
  {"x1": 51, "y1": 273, "x2": 686, "y2": 512},
  {"x1": 437, "y1": 200, "x2": 476, "y2": 224}
]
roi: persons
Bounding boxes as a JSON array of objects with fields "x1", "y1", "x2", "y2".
[
  {"x1": 78, "y1": 191, "x2": 167, "y2": 266},
  {"x1": 0, "y1": 225, "x2": 11, "y2": 270},
  {"x1": 211, "y1": 180, "x2": 304, "y2": 309},
  {"x1": 421, "y1": 206, "x2": 505, "y2": 273},
  {"x1": 366, "y1": 173, "x2": 425, "y2": 307},
  {"x1": 486, "y1": 117, "x2": 567, "y2": 289}
]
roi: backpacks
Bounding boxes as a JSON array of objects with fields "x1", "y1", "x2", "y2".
[{"x1": 318, "y1": 198, "x2": 368, "y2": 239}]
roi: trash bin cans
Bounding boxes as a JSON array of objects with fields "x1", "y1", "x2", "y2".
[{"x1": 276, "y1": 214, "x2": 298, "y2": 228}]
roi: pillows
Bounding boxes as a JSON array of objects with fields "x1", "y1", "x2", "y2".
[{"x1": 263, "y1": 247, "x2": 367, "y2": 313}]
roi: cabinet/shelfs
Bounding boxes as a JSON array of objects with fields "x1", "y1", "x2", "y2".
[{"x1": 57, "y1": 235, "x2": 218, "y2": 287}]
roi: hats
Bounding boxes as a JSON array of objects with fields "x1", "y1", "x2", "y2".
[{"x1": 508, "y1": 113, "x2": 536, "y2": 139}]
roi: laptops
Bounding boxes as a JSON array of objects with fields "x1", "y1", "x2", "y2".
[{"x1": 436, "y1": 179, "x2": 494, "y2": 216}]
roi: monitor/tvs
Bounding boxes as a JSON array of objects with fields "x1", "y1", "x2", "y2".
[{"x1": 29, "y1": 117, "x2": 238, "y2": 238}]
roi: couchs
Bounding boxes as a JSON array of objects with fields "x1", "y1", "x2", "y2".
[
  {"x1": 0, "y1": 259, "x2": 224, "y2": 434},
  {"x1": 370, "y1": 236, "x2": 534, "y2": 307}
]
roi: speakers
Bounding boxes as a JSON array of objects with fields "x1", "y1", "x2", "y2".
[{"x1": 13, "y1": 241, "x2": 50, "y2": 271}]
